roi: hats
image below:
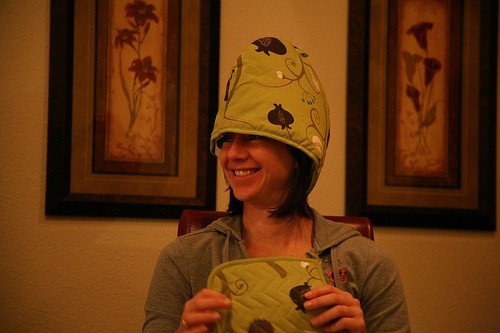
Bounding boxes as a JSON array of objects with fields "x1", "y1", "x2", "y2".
[{"x1": 210, "y1": 36, "x2": 329, "y2": 198}]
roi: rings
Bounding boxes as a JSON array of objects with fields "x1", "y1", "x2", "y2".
[{"x1": 178, "y1": 319, "x2": 187, "y2": 331}]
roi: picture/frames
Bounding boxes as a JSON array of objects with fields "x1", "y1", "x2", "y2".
[
  {"x1": 46, "y1": 0, "x2": 222, "y2": 221},
  {"x1": 344, "y1": 0, "x2": 500, "y2": 233}
]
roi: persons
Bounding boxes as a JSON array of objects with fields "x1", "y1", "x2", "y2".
[{"x1": 143, "y1": 36, "x2": 410, "y2": 333}]
